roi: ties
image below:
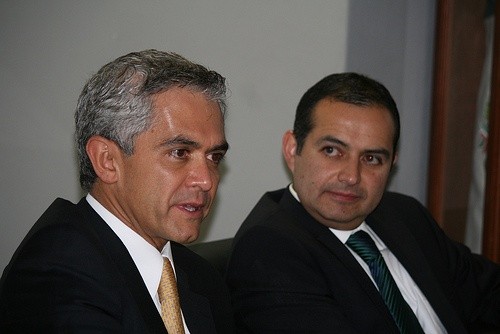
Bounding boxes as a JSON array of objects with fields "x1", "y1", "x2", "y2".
[
  {"x1": 158, "y1": 257, "x2": 186, "y2": 333},
  {"x1": 346, "y1": 231, "x2": 425, "y2": 334}
]
